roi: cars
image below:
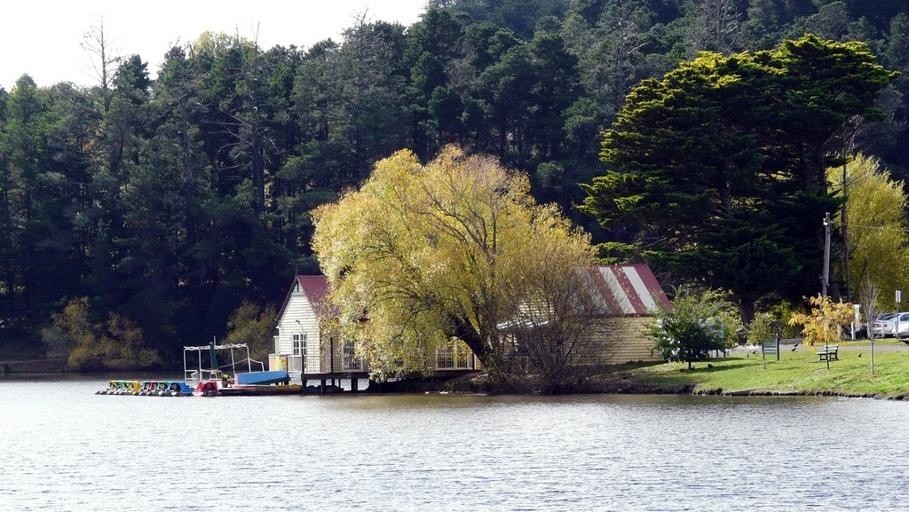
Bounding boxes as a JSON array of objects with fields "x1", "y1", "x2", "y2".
[{"x1": 843, "y1": 312, "x2": 909, "y2": 345}]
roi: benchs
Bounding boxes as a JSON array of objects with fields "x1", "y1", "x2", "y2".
[{"x1": 816, "y1": 343, "x2": 841, "y2": 363}]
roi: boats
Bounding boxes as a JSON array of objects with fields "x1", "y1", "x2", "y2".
[{"x1": 94, "y1": 369, "x2": 302, "y2": 394}]
